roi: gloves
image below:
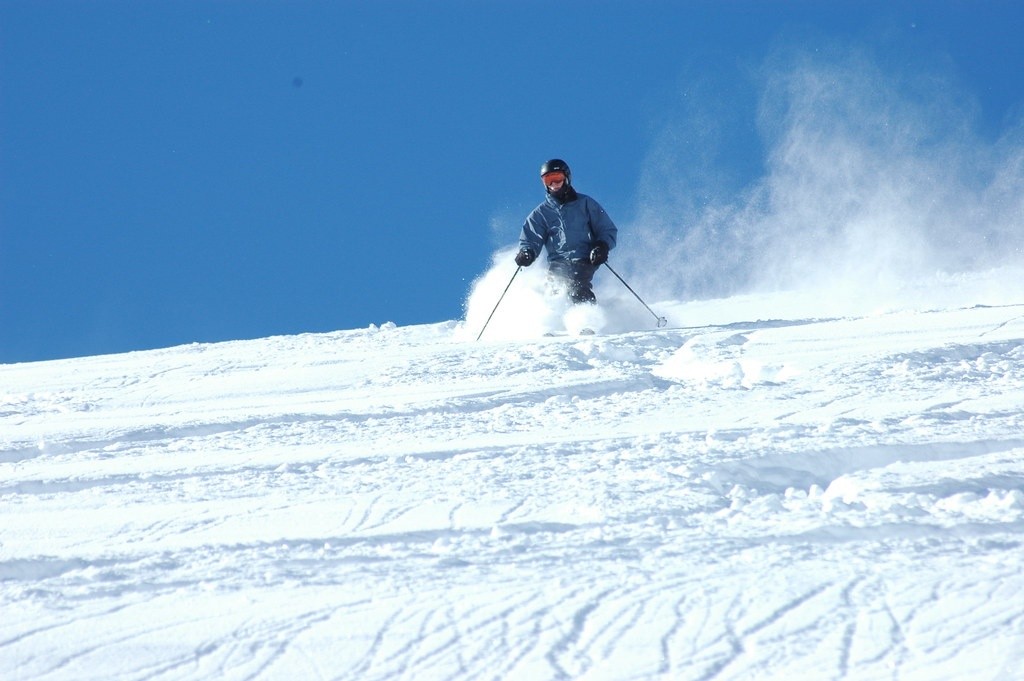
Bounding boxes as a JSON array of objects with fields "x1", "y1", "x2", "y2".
[
  {"x1": 515, "y1": 249, "x2": 535, "y2": 266},
  {"x1": 590, "y1": 240, "x2": 608, "y2": 266}
]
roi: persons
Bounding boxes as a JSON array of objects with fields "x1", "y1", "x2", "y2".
[{"x1": 515, "y1": 159, "x2": 617, "y2": 304}]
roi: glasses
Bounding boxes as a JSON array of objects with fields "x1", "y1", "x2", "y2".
[{"x1": 542, "y1": 171, "x2": 565, "y2": 185}]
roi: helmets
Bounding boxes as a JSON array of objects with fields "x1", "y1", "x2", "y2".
[{"x1": 541, "y1": 159, "x2": 571, "y2": 184}]
